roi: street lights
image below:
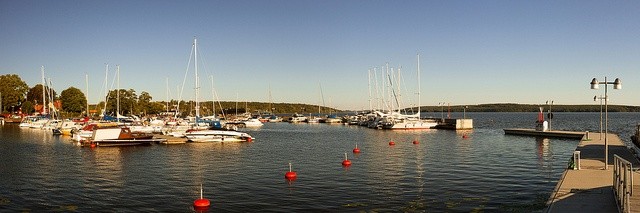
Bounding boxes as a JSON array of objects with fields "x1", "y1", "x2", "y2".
[
  {"x1": 546, "y1": 100, "x2": 553, "y2": 130},
  {"x1": 594, "y1": 96, "x2": 608, "y2": 140},
  {"x1": 591, "y1": 77, "x2": 622, "y2": 170}
]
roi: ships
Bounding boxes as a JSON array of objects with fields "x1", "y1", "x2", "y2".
[
  {"x1": 154, "y1": 38, "x2": 213, "y2": 137},
  {"x1": 72, "y1": 66, "x2": 154, "y2": 142},
  {"x1": 52, "y1": 74, "x2": 83, "y2": 134},
  {"x1": 29, "y1": 65, "x2": 48, "y2": 128},
  {"x1": 42, "y1": 81, "x2": 72, "y2": 129}
]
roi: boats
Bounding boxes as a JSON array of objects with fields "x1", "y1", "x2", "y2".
[
  {"x1": 183, "y1": 128, "x2": 255, "y2": 142},
  {"x1": 288, "y1": 112, "x2": 308, "y2": 123},
  {"x1": 325, "y1": 118, "x2": 342, "y2": 123},
  {"x1": 348, "y1": 115, "x2": 359, "y2": 125},
  {"x1": 269, "y1": 116, "x2": 283, "y2": 123},
  {"x1": 308, "y1": 116, "x2": 324, "y2": 123},
  {"x1": 19, "y1": 116, "x2": 44, "y2": 127},
  {"x1": 245, "y1": 118, "x2": 264, "y2": 127}
]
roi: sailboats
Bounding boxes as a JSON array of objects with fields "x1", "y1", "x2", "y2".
[{"x1": 359, "y1": 54, "x2": 442, "y2": 129}]
